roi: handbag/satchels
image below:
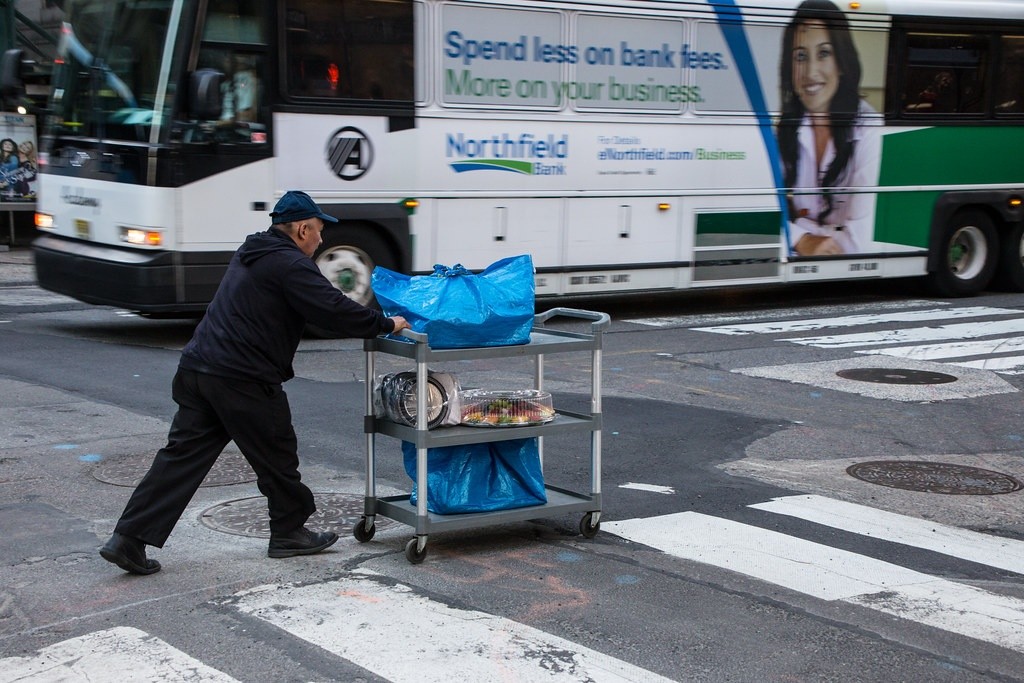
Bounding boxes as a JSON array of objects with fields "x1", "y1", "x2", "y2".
[
  {"x1": 370, "y1": 255, "x2": 538, "y2": 351},
  {"x1": 398, "y1": 434, "x2": 549, "y2": 512}
]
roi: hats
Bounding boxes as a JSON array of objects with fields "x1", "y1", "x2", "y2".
[{"x1": 267, "y1": 189, "x2": 338, "y2": 226}]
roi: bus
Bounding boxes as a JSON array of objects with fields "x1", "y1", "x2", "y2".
[{"x1": 4, "y1": 1, "x2": 1024, "y2": 339}]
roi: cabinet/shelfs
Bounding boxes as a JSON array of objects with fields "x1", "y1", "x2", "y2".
[{"x1": 352, "y1": 308, "x2": 609, "y2": 562}]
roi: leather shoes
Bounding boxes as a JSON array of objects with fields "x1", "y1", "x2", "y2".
[
  {"x1": 99, "y1": 529, "x2": 161, "y2": 576},
  {"x1": 267, "y1": 521, "x2": 340, "y2": 559}
]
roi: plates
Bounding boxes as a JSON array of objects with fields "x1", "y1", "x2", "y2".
[{"x1": 464, "y1": 416, "x2": 553, "y2": 428}]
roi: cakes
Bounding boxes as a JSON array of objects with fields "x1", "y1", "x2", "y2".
[{"x1": 462, "y1": 397, "x2": 554, "y2": 425}]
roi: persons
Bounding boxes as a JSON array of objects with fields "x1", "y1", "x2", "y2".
[
  {"x1": 100, "y1": 191, "x2": 412, "y2": 573},
  {"x1": 0, "y1": 138, "x2": 37, "y2": 196},
  {"x1": 201, "y1": 51, "x2": 257, "y2": 137},
  {"x1": 777, "y1": 0, "x2": 885, "y2": 257}
]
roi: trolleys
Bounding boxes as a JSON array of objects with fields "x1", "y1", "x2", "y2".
[{"x1": 352, "y1": 306, "x2": 612, "y2": 563}]
